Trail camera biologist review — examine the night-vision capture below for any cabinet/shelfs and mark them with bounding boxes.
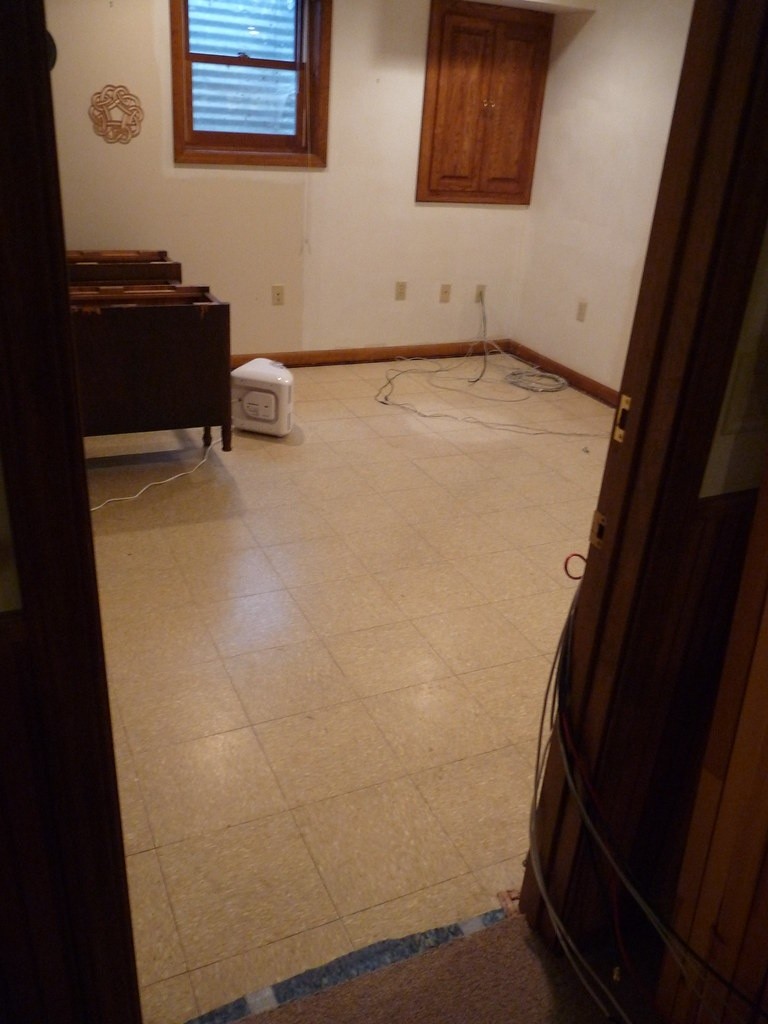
[67,248,234,454]
[414,0,555,206]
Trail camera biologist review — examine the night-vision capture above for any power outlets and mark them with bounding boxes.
[397,282,406,301]
[439,284,451,303]
[273,284,285,306]
[576,300,587,321]
[476,284,486,302]
